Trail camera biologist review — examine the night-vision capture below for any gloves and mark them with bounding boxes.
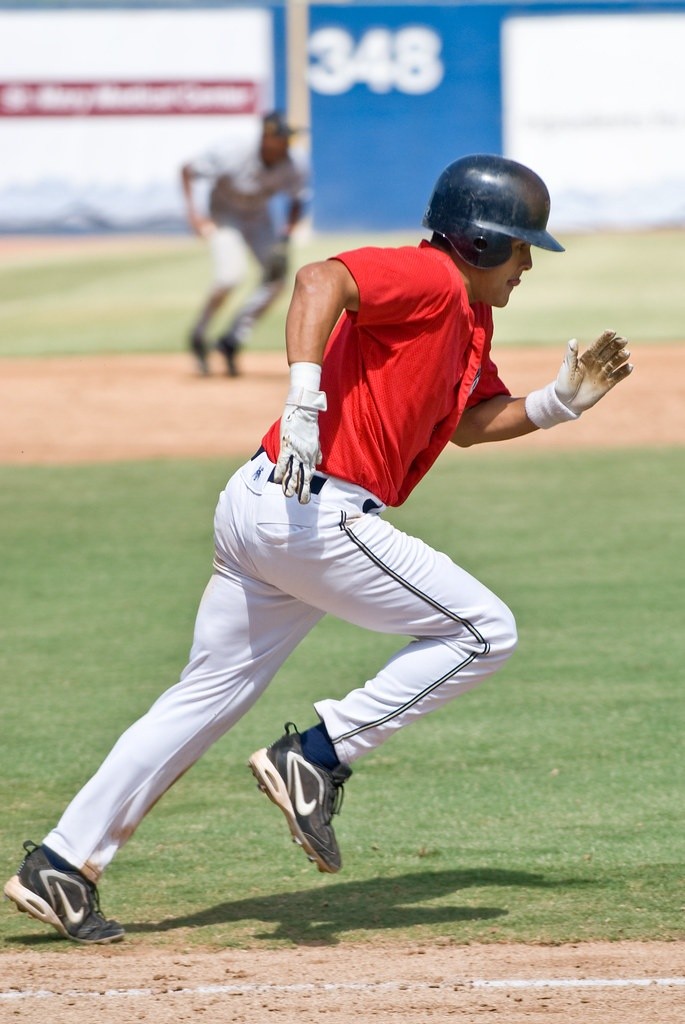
[554,330,633,414]
[274,383,327,504]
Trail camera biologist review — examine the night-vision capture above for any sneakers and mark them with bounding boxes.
[3,840,125,944]
[246,721,352,873]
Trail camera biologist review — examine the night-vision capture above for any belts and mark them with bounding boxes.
[250,446,382,517]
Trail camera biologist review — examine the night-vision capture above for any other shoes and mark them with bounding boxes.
[188,330,212,377]
[220,336,242,376]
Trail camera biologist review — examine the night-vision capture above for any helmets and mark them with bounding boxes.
[421,155,566,269]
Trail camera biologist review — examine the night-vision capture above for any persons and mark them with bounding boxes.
[177,112,312,379]
[0,158,635,948]
